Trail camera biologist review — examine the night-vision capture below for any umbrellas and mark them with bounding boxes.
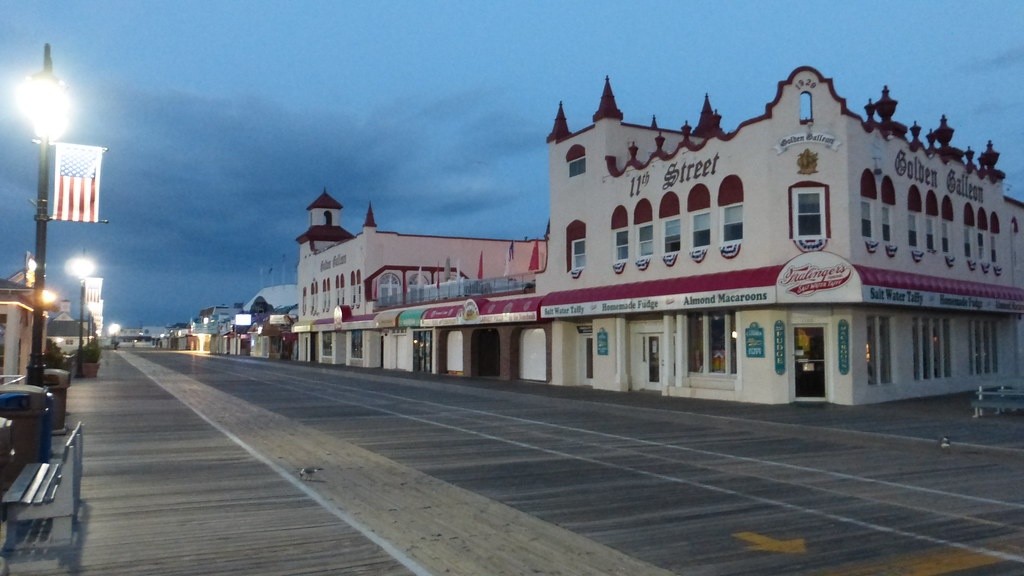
[528,237,539,270]
[371,255,461,301]
[478,250,482,279]
[504,241,513,276]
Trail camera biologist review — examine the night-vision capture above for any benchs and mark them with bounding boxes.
[0,422,84,550]
[970,384,1024,418]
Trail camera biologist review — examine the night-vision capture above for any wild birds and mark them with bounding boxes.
[299,467,324,481]
[940,436,950,448]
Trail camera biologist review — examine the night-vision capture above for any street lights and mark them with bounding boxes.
[73,248,90,380]
[18,44,69,386]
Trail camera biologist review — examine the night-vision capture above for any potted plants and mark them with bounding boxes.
[82,338,101,379]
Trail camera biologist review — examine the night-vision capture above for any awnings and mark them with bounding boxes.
[775,251,1023,314]
[290,296,544,334]
[539,265,783,319]
